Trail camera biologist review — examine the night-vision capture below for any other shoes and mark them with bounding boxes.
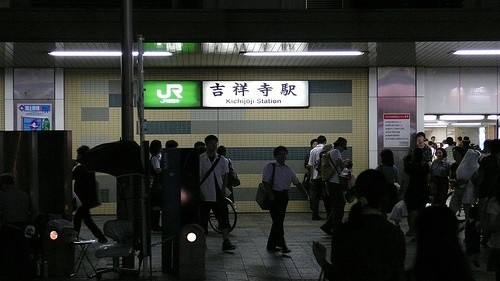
[267,246,280,251]
[406,231,416,237]
[319,225,332,235]
[221,241,236,250]
[282,247,291,253]
[312,216,325,220]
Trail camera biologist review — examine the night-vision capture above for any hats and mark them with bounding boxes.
[334,137,347,150]
[462,136,470,142]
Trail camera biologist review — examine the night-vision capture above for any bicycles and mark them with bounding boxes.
[209,197,237,234]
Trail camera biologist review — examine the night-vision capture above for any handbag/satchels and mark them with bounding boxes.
[389,200,408,223]
[255,183,273,210]
[302,174,311,193]
[461,181,478,204]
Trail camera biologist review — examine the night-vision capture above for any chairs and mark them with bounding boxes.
[73,219,133,281]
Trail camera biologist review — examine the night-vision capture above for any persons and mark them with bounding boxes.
[145,135,239,251]
[72,146,108,243]
[261,146,308,253]
[303,132,500,281]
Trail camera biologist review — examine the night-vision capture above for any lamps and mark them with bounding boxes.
[447,48,500,56]
[46,49,174,58]
[422,114,500,127]
[238,49,369,58]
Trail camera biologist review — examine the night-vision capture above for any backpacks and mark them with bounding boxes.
[321,149,338,180]
